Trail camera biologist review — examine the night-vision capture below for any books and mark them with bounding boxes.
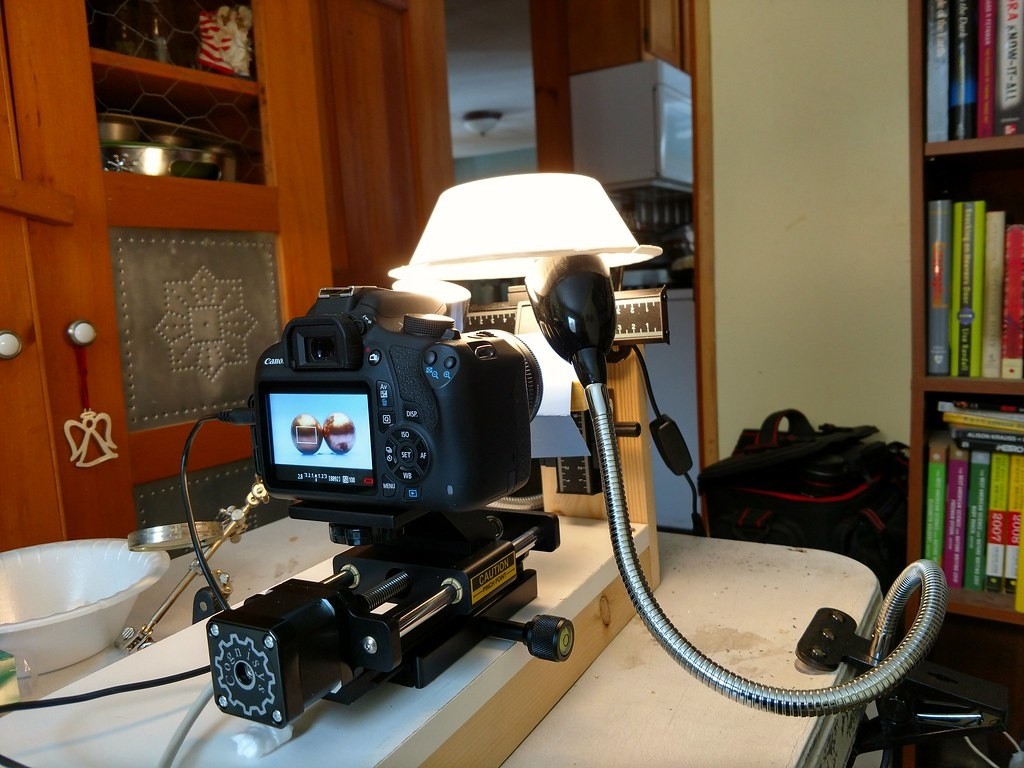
[914,0,1024,618]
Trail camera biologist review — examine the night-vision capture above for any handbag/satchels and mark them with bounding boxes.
[701,403,910,601]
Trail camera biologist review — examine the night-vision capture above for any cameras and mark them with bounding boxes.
[248,287,543,511]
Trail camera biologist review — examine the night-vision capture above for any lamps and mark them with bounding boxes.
[463,110,503,138]
[388,173,664,307]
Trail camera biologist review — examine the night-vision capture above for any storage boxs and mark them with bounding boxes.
[697,408,910,639]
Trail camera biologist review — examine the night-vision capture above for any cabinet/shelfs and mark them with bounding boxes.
[565,0,691,77]
[0,0,454,555]
[905,0,1023,641]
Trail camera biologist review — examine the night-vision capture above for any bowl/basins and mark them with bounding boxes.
[101,108,257,182]
[1,537,173,680]
